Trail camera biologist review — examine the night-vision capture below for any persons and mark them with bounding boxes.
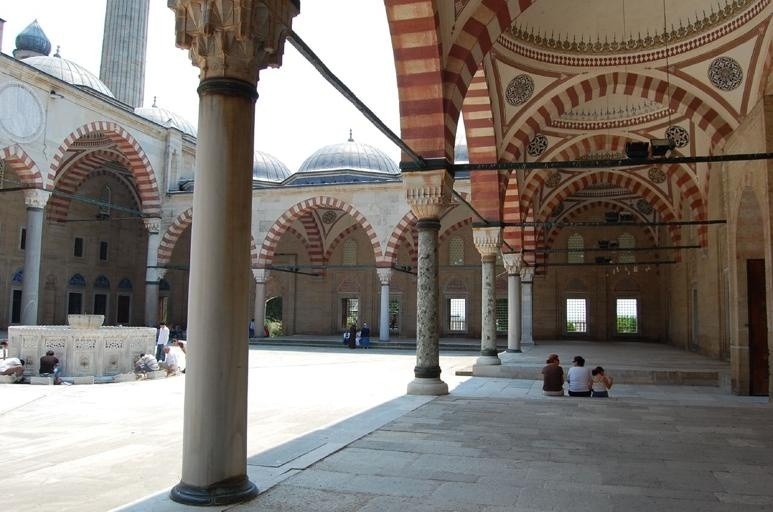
[588,366,615,397]
[541,352,564,396]
[0,342,8,360]
[390,314,397,330]
[343,321,370,348]
[264,323,269,336]
[0,357,26,383]
[249,319,254,338]
[134,321,187,376]
[39,350,62,385]
[566,356,593,397]
